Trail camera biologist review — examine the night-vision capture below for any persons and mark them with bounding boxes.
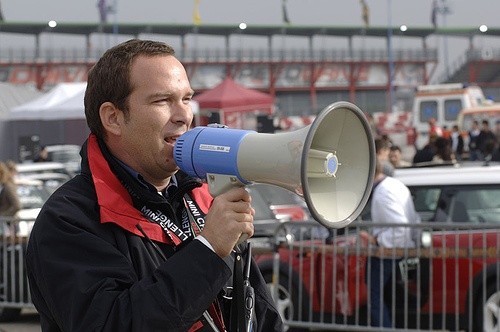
[357,153,423,329]
[34,145,53,161]
[26,38,282,331]
[366,118,499,175]
[0,159,21,237]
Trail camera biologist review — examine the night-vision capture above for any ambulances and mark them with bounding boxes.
[458,102,500,136]
[413,83,488,137]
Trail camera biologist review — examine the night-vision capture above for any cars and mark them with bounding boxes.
[248,182,311,220]
[43,144,82,173]
[0,160,72,320]
[232,187,277,224]
[249,169,500,332]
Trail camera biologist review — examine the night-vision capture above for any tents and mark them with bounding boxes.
[1,78,93,164]
[193,74,273,135]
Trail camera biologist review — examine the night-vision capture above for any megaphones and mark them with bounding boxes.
[173,101,376,230]
